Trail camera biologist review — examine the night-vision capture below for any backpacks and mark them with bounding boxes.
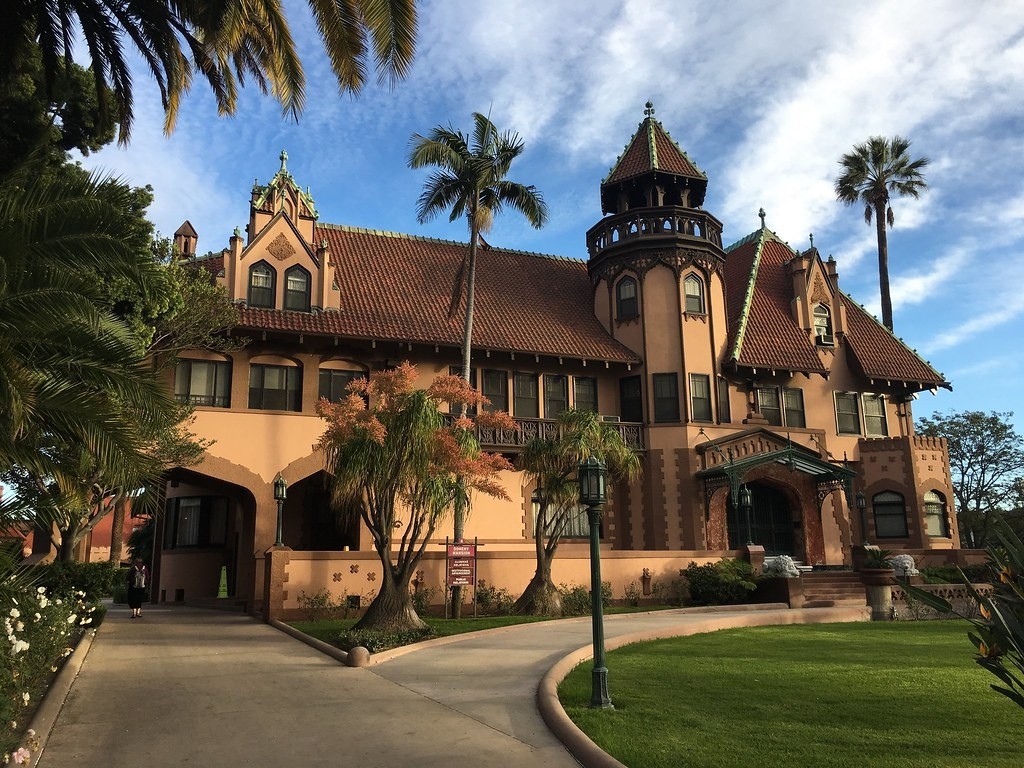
[134,566,146,588]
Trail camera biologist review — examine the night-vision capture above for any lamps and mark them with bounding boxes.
[740,483,752,507]
[785,461,797,473]
[274,477,288,500]
[856,487,867,509]
[578,455,606,505]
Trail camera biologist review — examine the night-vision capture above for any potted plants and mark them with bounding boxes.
[858,549,897,586]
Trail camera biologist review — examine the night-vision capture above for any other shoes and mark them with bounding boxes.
[130,615,136,619]
[136,614,142,617]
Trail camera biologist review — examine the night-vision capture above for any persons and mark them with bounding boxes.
[126,556,150,618]
